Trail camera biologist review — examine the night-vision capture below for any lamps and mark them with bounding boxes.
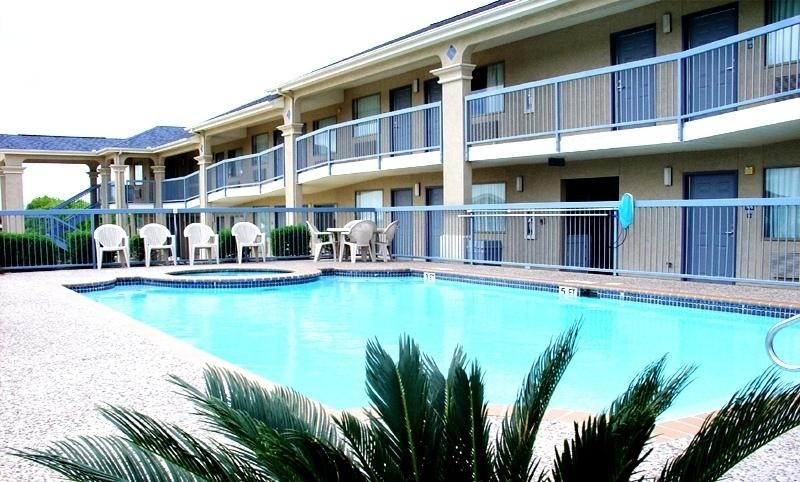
[664,168,672,187]
[515,176,523,191]
[414,183,419,196]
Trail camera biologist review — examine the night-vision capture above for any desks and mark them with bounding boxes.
[327,227,387,262]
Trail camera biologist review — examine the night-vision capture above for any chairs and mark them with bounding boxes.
[338,220,376,263]
[184,223,221,267]
[343,219,362,229]
[231,221,266,265]
[95,224,131,269]
[306,220,337,262]
[139,223,176,269]
[369,220,399,262]
